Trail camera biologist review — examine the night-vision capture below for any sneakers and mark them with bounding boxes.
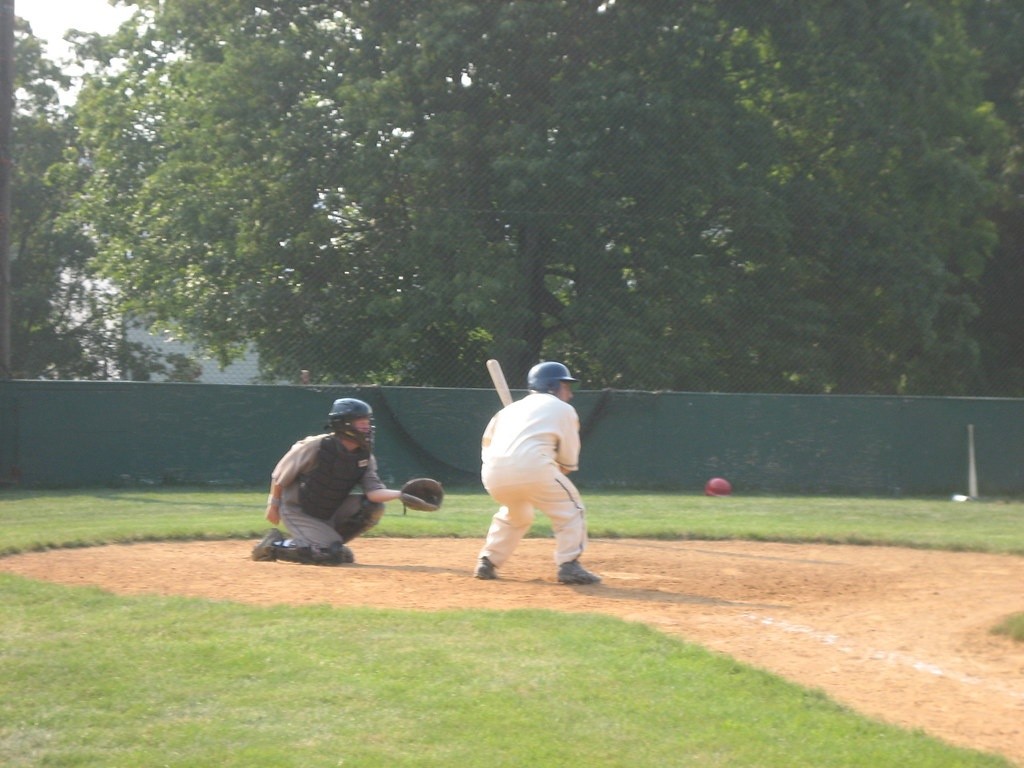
[474,559,497,580]
[331,544,355,564]
[251,529,283,561]
[557,560,602,583]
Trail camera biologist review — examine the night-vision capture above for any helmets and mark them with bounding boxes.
[527,362,579,397]
[329,398,375,456]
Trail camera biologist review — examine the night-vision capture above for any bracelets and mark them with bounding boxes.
[268,494,283,505]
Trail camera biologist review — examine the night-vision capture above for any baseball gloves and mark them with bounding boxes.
[401,479,445,512]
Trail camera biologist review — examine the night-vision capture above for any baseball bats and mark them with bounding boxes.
[486,359,514,408]
[966,423,979,499]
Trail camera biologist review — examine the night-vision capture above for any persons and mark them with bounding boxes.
[474,361,603,583]
[251,397,439,566]
